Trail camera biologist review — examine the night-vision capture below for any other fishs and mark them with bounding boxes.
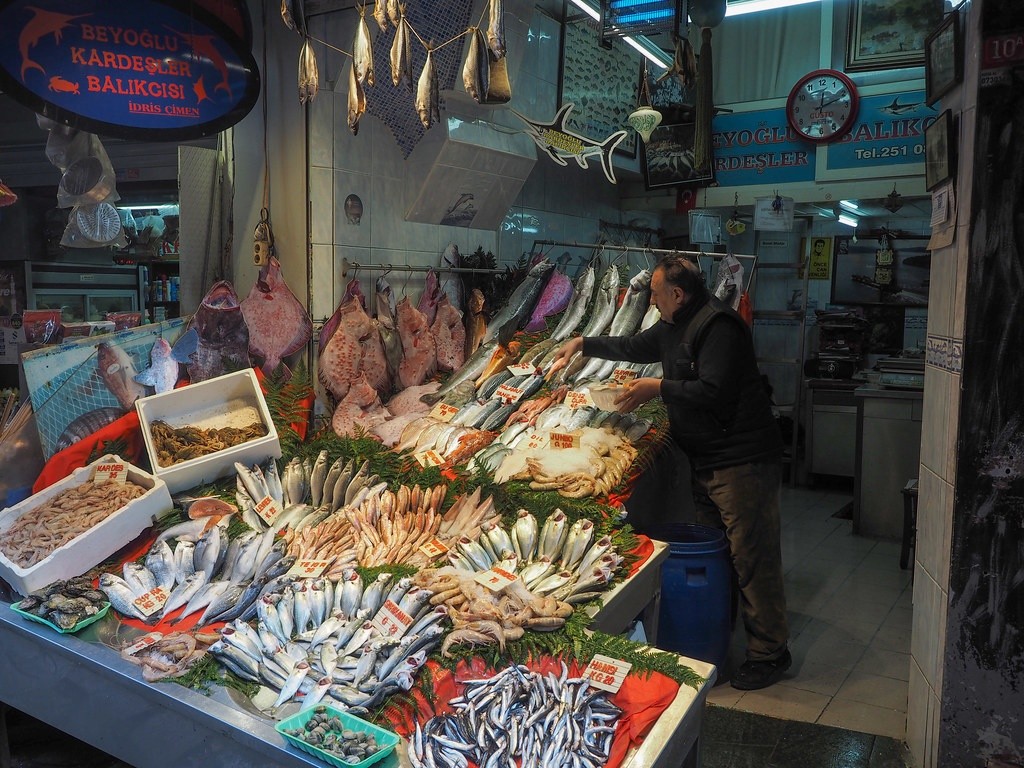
[97,505,297,626]
[298,569,447,715]
[207,619,299,713]
[320,251,745,443]
[402,665,624,768]
[279,0,507,137]
[646,140,707,180]
[233,443,526,567]
[527,508,626,602]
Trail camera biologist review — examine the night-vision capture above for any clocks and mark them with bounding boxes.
[786,68,861,146]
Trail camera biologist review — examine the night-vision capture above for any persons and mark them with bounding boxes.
[555,256,794,691]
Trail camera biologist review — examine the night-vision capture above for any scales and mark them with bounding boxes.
[877,357,925,390]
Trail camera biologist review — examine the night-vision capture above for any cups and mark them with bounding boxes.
[153,279,170,304]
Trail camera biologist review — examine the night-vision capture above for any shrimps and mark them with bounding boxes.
[526,445,638,499]
[111,631,220,683]
[1,471,149,563]
[415,568,574,659]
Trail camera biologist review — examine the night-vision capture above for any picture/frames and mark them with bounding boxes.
[923,107,953,192]
[923,10,962,108]
[637,115,718,193]
[842,0,944,74]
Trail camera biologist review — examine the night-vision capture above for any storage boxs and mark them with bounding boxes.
[0,453,174,600]
[135,366,284,494]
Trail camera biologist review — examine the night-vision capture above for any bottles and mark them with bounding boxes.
[144,267,150,305]
[162,273,168,303]
[170,273,179,302]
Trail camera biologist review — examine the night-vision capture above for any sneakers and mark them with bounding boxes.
[730,648,797,690]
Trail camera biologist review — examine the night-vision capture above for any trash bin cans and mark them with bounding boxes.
[633,520,734,689]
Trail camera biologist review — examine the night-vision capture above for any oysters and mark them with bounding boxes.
[18,576,104,632]
